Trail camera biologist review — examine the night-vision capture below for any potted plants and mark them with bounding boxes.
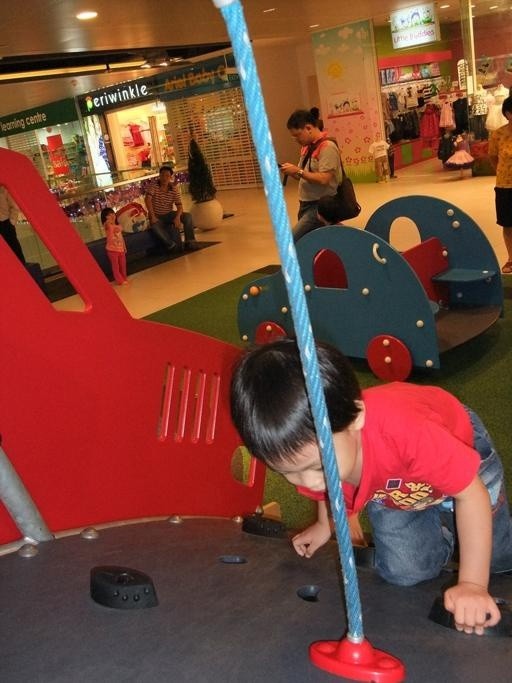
[185,137,224,231]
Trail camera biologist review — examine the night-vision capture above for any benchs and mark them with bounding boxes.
[87,222,182,276]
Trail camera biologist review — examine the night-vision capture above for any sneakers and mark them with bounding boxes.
[171,245,179,253]
[184,241,198,250]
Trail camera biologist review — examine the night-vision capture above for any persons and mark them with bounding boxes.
[311,107,323,132]
[488,97,512,274]
[227,330,511,636]
[1,185,26,267]
[317,196,344,225]
[143,165,198,253]
[368,134,391,182]
[385,137,397,179]
[482,83,512,132]
[277,108,344,247]
[100,206,130,286]
[446,134,475,169]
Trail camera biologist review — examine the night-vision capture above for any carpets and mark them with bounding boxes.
[44,239,221,306]
[137,263,511,541]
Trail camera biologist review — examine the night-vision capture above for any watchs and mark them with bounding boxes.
[294,168,304,180]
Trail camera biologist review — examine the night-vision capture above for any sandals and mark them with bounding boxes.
[502,261,512,273]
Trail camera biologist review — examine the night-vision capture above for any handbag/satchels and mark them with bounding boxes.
[332,177,361,219]
